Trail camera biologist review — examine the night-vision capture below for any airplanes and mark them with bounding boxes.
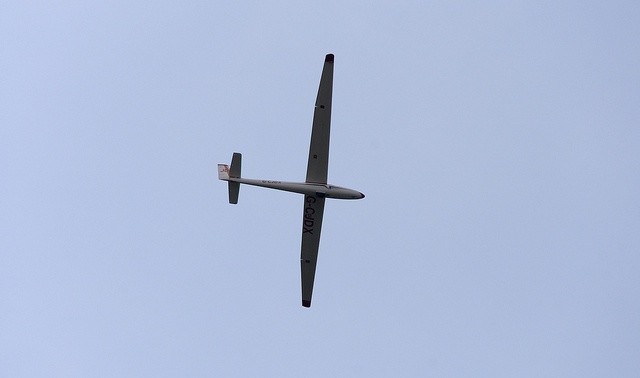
[217,54,366,308]
